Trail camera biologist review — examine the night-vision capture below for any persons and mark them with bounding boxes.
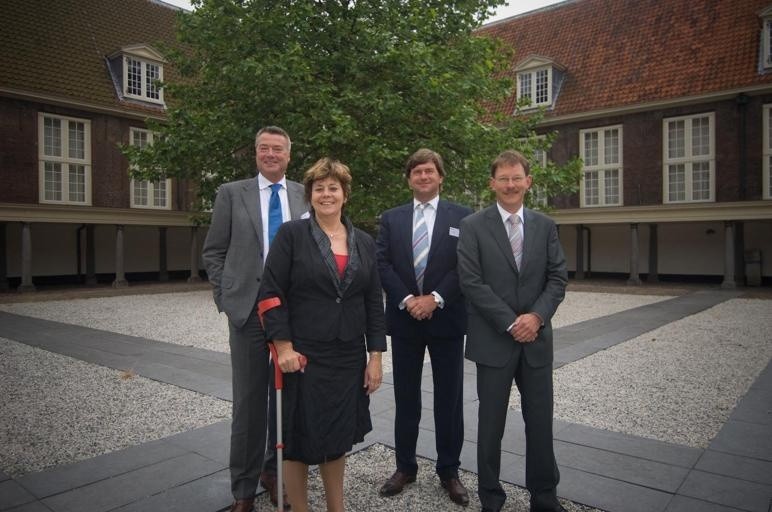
[375,148,472,508]
[454,150,569,511]
[200,125,311,510]
[256,159,385,510]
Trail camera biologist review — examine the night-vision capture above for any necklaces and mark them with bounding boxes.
[321,223,340,240]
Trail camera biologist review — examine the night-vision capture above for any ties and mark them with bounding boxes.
[412,203,431,294]
[268,184,282,248]
[508,214,523,272]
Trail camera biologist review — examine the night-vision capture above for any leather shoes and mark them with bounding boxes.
[230,478,291,512]
[380,471,416,496]
[441,477,469,506]
